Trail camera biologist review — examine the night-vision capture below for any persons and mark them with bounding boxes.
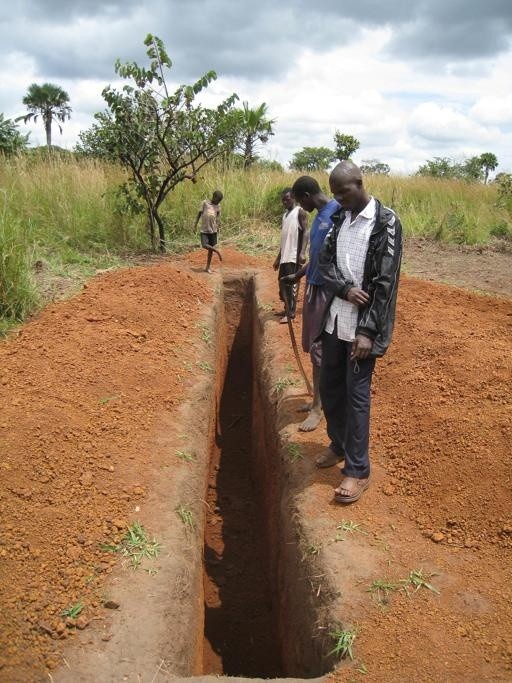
[315,162,403,503]
[279,175,343,432]
[273,187,309,323]
[193,190,224,274]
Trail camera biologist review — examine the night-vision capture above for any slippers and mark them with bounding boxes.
[316,449,346,468]
[332,475,371,503]
